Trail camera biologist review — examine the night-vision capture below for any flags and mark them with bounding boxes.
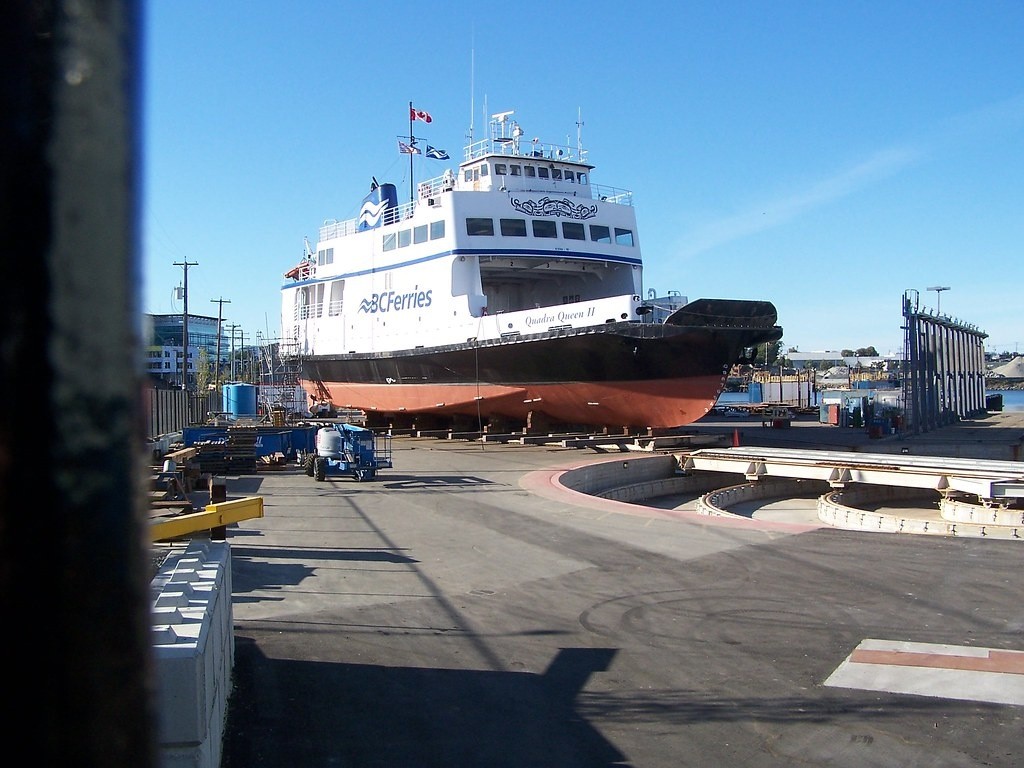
[425,144,450,161]
[397,141,422,155]
[411,108,433,125]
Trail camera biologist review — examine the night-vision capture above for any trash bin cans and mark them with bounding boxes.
[828,403,840,424]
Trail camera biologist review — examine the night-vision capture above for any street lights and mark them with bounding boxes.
[924,286,951,313]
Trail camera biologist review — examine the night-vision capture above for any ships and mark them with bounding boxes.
[274,99,787,430]
